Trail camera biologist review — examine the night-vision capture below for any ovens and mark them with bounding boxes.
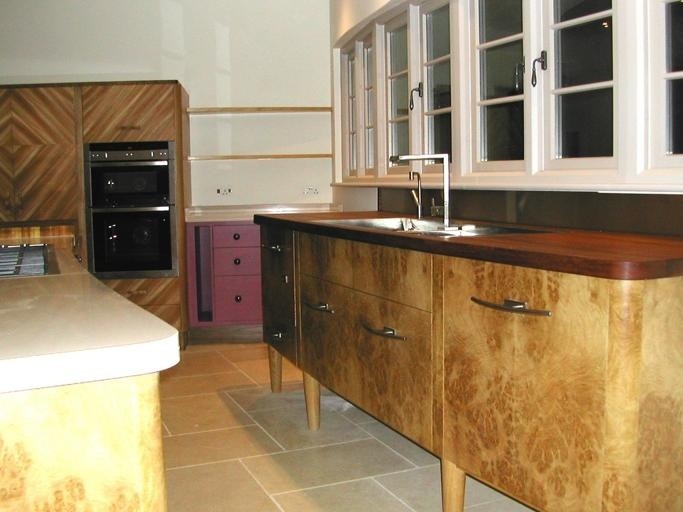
[83,140,180,279]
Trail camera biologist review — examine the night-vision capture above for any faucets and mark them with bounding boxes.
[390,153,450,230]
[409,172,422,219]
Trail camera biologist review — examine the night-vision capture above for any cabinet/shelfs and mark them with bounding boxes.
[80,82,189,143]
[0,82,76,229]
[260,226,297,367]
[97,277,181,334]
[186,221,260,328]
[185,105,333,161]
[443,256,682,510]
[296,231,433,454]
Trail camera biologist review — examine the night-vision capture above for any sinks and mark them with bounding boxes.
[418,224,551,236]
[318,217,442,229]
